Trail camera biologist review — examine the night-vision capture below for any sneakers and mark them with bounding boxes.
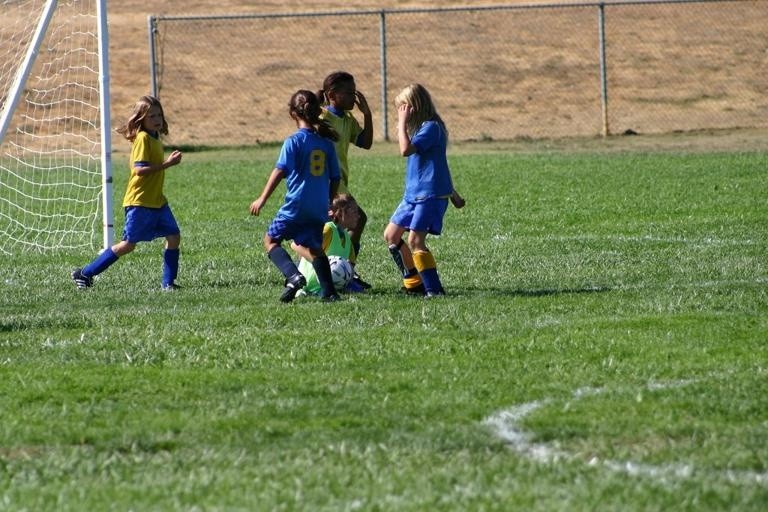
[279,272,371,302]
[162,284,183,289]
[398,283,445,299]
[71,269,93,290]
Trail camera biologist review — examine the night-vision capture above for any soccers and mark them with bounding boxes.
[316,255,352,290]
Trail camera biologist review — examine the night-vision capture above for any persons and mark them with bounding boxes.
[290,192,361,298]
[314,72,373,290]
[71,96,184,291]
[383,83,465,298]
[250,90,342,303]
